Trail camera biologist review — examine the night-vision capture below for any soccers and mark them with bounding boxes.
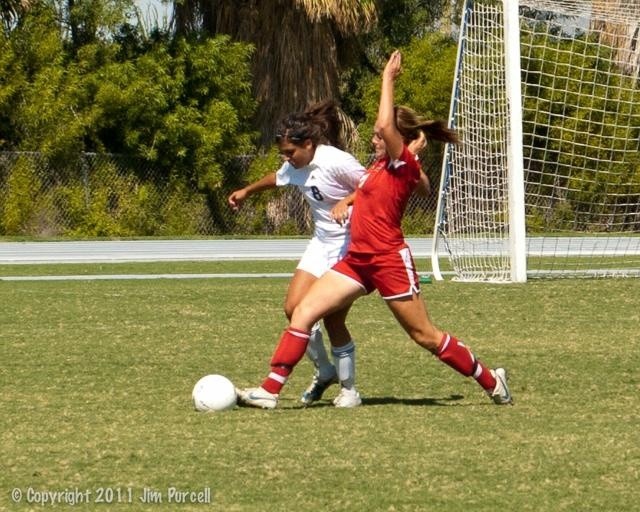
[191,375,237,414]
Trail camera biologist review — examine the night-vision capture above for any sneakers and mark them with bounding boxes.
[300,365,362,409]
[236,386,279,410]
[491,368,511,405]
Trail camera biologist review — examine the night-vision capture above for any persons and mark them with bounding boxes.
[234,46,515,416]
[225,98,365,411]
